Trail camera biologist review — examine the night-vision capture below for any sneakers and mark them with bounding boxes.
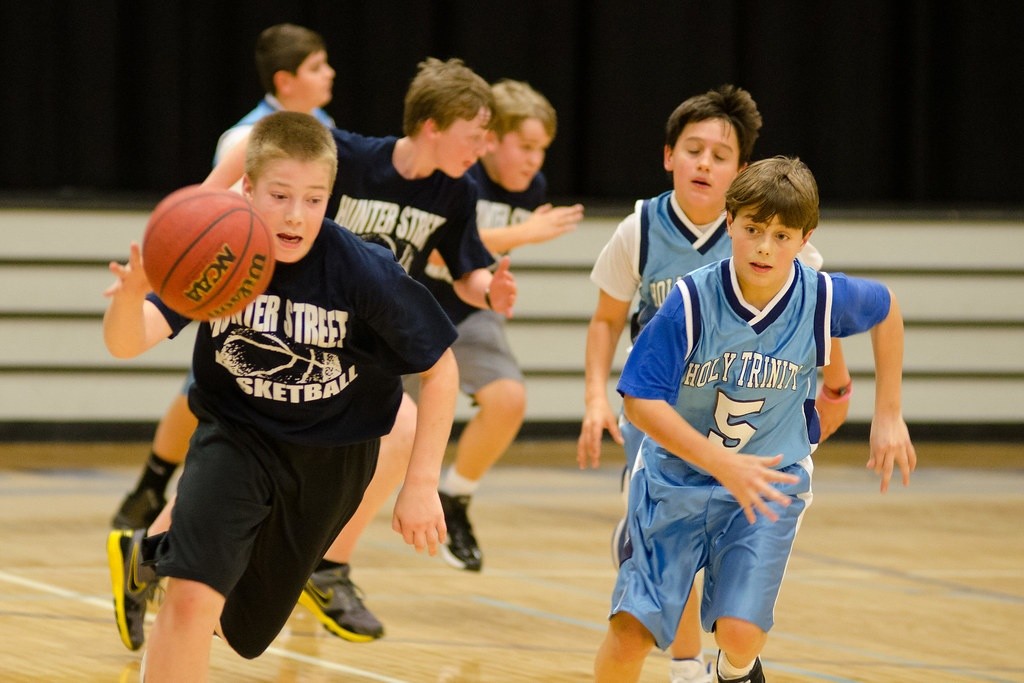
[437,488,482,571]
[106,529,167,653]
[297,564,385,644]
[112,489,166,538]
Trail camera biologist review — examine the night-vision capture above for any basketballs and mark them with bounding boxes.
[140,184,277,322]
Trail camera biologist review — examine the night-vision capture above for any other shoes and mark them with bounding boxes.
[668,658,715,683]
[612,465,633,570]
[715,649,766,683]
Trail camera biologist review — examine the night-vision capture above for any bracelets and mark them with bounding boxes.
[822,380,851,403]
[485,288,491,309]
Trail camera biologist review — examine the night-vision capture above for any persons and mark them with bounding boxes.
[102,114,460,683]
[113,24,337,530]
[574,87,852,683]
[106,61,516,651]
[592,159,917,683]
[404,82,583,572]
